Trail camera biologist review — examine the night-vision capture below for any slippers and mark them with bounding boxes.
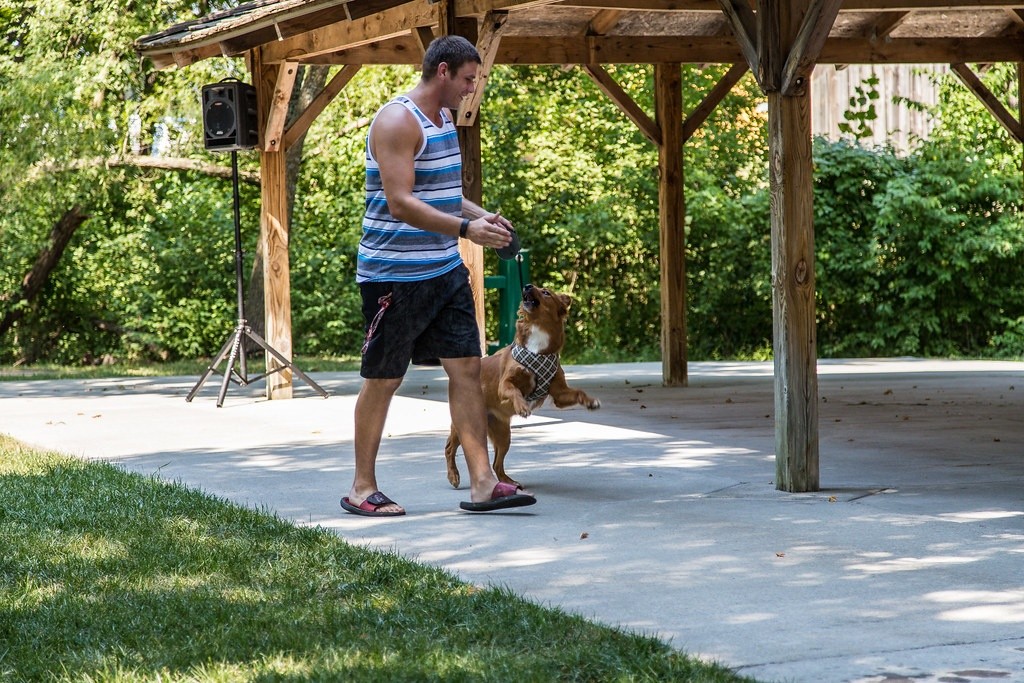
[460,481,537,511]
[340,492,406,517]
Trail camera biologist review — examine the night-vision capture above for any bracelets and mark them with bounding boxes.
[459,218,471,238]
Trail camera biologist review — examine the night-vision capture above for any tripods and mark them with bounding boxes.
[185,148,331,407]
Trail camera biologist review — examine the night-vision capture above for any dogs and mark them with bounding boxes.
[445,283,601,489]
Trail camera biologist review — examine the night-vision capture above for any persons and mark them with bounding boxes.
[340,36,537,516]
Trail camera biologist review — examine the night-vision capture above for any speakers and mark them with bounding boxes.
[202,83,259,149]
